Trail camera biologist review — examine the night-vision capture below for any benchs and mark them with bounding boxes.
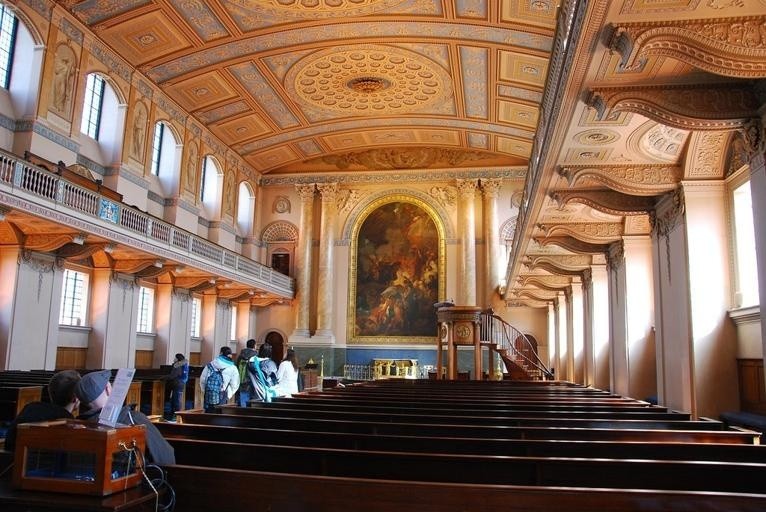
[0,366,766,512]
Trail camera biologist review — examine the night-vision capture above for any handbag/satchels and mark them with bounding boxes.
[298,374,304,392]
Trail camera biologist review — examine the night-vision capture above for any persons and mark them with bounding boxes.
[5,368,80,450]
[237,339,258,406]
[199,346,241,408]
[276,348,301,397]
[166,353,189,413]
[246,344,279,401]
[74,370,177,466]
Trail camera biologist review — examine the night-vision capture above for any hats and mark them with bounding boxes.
[75,369,112,404]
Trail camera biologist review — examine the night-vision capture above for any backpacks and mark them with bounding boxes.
[238,354,256,392]
[204,363,231,412]
[175,363,188,384]
